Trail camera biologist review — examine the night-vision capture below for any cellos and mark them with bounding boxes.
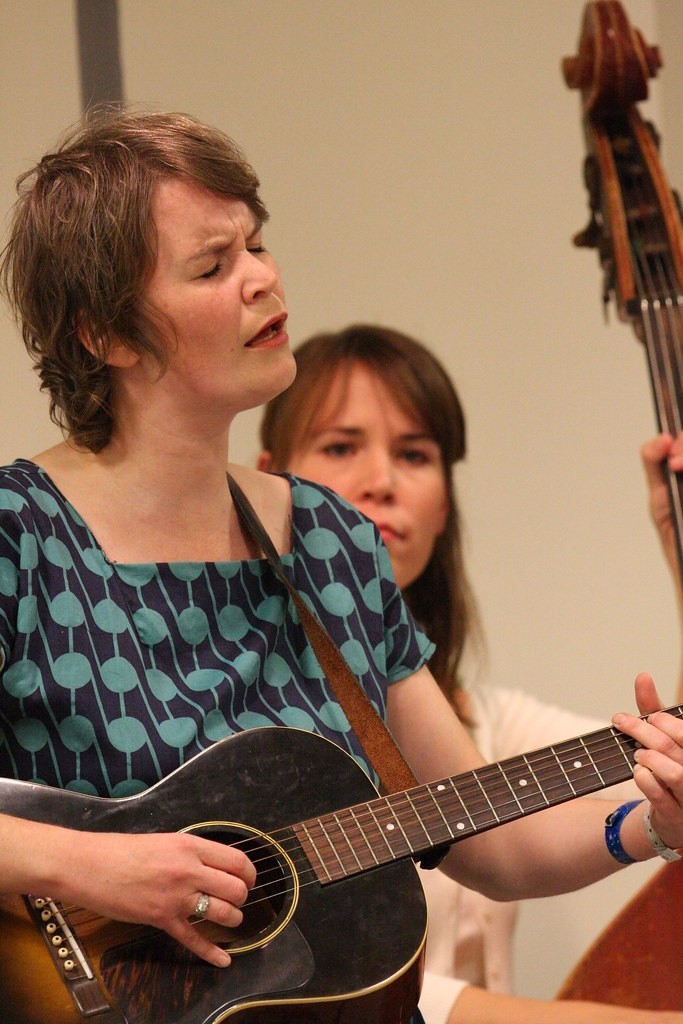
[553,0,683,1013]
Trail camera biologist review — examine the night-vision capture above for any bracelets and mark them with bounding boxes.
[602,796,648,864]
[642,804,682,863]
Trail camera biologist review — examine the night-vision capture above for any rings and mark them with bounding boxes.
[193,893,209,918]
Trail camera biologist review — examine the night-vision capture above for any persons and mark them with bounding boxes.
[255,326,683,1024]
[0,110,683,1024]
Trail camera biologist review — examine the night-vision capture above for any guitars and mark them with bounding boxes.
[1,700,682,1024]
